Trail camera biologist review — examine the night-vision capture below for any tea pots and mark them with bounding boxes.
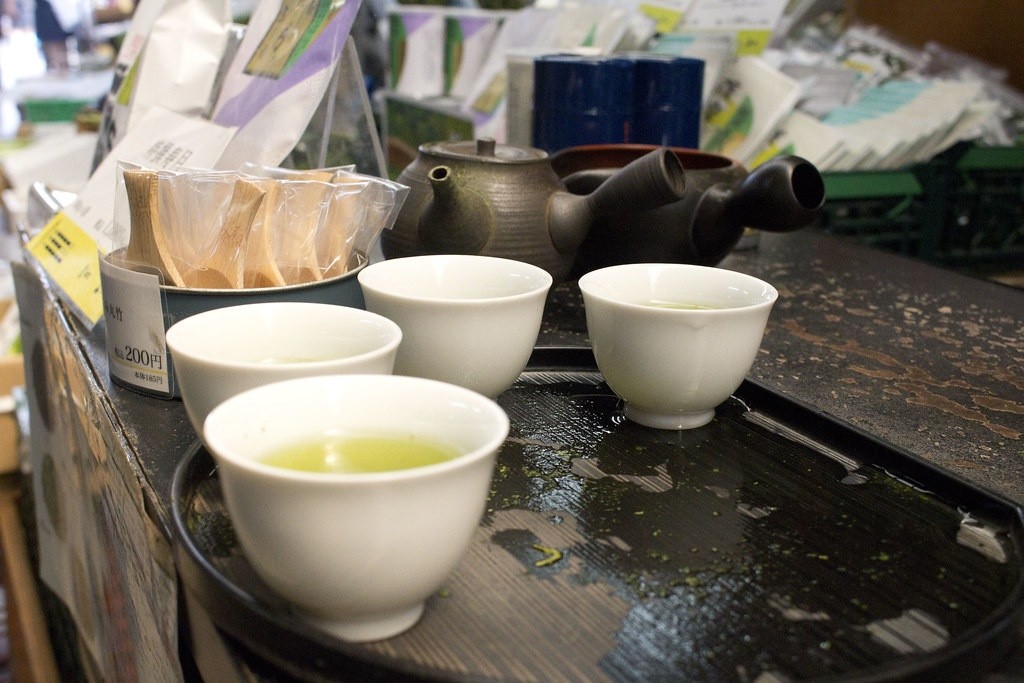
[380,136,688,286]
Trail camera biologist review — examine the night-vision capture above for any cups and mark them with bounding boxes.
[356,255,553,405]
[165,302,403,457]
[505,52,704,157]
[204,375,510,643]
[577,262,779,431]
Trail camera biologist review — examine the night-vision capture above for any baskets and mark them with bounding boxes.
[817,144,1024,271]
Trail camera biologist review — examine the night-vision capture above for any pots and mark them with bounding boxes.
[555,145,826,269]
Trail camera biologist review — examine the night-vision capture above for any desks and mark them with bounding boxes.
[14,228,1024,683]
